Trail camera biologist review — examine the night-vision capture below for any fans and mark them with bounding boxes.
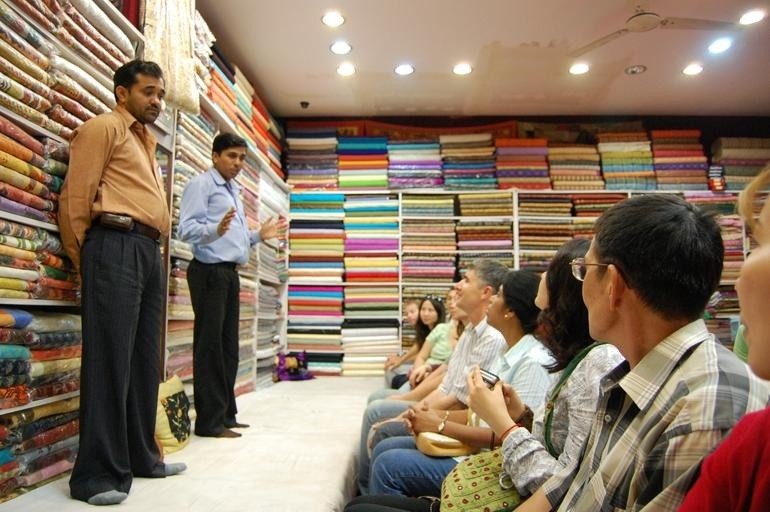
[568,0,747,57]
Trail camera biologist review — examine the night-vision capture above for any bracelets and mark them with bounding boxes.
[518,406,534,427]
[444,409,450,420]
[439,419,445,433]
[500,424,519,442]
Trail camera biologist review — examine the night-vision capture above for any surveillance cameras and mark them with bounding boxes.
[300,102,309,109]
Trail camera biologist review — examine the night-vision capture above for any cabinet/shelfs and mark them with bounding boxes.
[288,189,770,377]
[0,0,287,503]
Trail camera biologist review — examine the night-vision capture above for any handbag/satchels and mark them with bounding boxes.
[439,446,529,511]
[416,431,481,458]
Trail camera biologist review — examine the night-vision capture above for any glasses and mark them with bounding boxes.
[425,294,442,302]
[570,257,611,282]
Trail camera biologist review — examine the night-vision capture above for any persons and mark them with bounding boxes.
[368,295,470,403]
[370,270,559,499]
[343,237,626,511]
[674,161,769,511]
[514,193,770,512]
[58,59,187,506]
[177,133,290,439]
[357,259,510,494]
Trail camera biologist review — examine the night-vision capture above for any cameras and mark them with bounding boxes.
[479,367,499,385]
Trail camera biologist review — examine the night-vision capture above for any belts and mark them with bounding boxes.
[91,213,164,246]
[215,260,242,271]
